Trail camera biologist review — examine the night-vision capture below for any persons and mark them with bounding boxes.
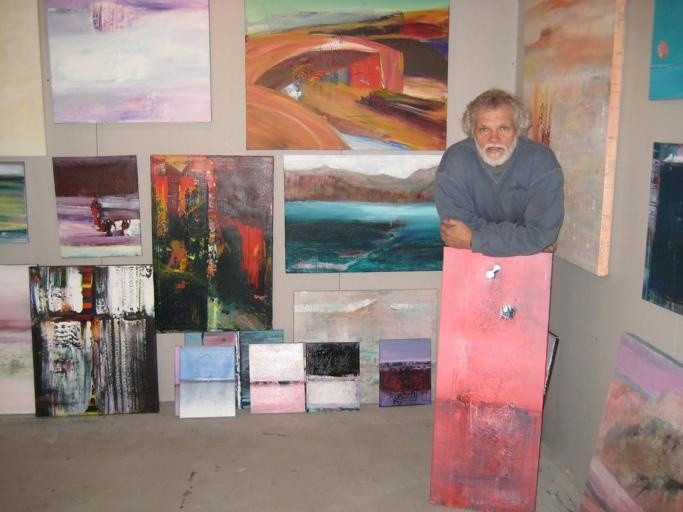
[433,89,564,257]
